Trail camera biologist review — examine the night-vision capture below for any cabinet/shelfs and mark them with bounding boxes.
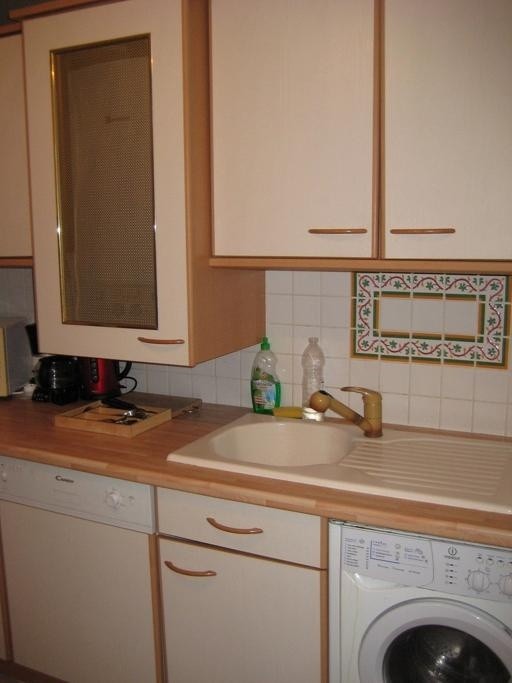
[8,0,265,367]
[208,0,512,275]
[156,487,331,683]
[1,454,164,682]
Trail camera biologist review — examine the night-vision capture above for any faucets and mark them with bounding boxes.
[306,386,384,438]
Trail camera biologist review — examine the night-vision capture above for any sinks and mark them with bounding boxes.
[167,410,353,484]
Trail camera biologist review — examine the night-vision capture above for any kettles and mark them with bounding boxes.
[77,357,132,401]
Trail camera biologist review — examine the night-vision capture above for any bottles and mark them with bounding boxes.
[301,336,325,409]
[251,336,282,415]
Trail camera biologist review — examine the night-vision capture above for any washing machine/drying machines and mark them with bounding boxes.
[328,517,512,683]
